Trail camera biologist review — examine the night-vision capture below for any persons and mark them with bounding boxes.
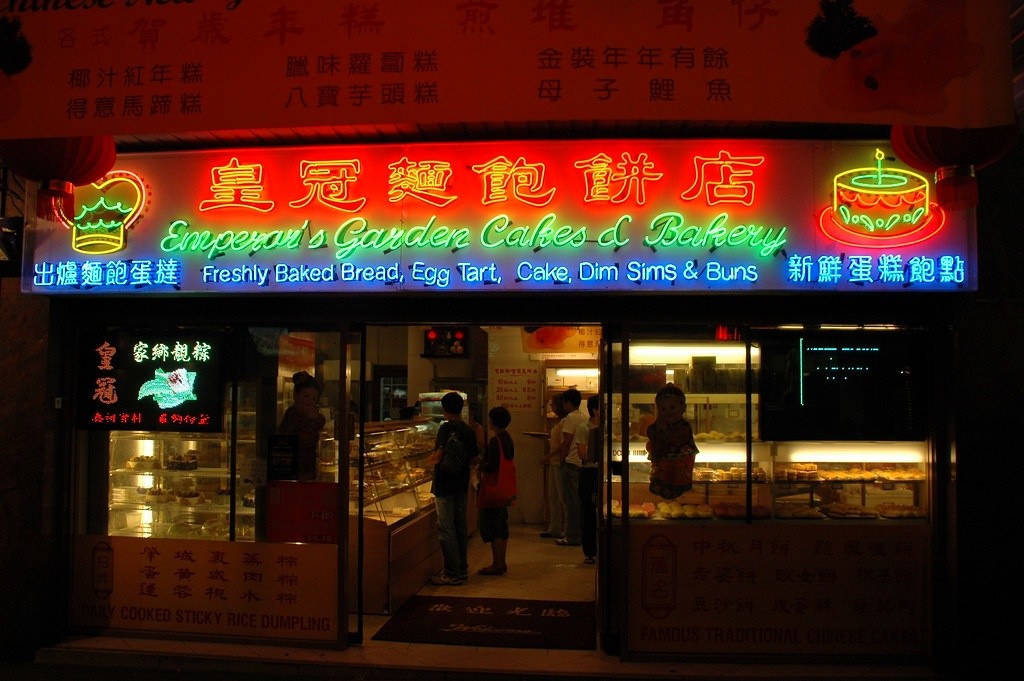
[538,389,606,565]
[425,391,480,585]
[475,407,515,576]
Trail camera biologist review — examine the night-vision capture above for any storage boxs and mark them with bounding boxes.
[616,365,667,393]
[675,357,759,394]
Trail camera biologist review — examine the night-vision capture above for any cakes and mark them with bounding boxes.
[125,452,256,508]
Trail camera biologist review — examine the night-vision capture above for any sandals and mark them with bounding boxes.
[584,554,597,564]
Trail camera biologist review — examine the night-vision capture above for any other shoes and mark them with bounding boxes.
[555,538,581,546]
[540,531,561,538]
[430,570,468,585]
[479,563,507,576]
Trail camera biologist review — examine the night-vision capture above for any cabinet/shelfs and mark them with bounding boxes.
[543,359,762,531]
[108,417,486,615]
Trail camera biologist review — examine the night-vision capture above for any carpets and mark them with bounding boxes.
[371,595,597,651]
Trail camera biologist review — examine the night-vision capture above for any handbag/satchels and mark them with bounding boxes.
[476,437,517,509]
[439,421,467,473]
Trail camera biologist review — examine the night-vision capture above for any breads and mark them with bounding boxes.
[774,463,926,520]
[601,498,770,518]
[349,438,437,516]
[692,431,760,442]
[691,465,767,482]
[611,432,648,442]
